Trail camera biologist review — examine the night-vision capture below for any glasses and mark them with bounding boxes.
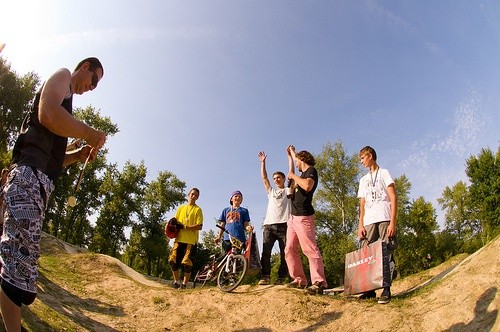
[92,67,99,88]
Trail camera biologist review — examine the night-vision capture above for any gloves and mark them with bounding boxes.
[174,221,186,230]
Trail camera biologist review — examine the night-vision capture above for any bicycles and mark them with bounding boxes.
[193,224,248,292]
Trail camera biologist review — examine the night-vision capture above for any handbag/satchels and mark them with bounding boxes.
[244,229,263,276]
[344,236,390,295]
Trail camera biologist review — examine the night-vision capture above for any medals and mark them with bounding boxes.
[68,196,77,207]
[372,188,377,193]
[185,218,188,221]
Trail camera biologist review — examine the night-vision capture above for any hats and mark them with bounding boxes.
[229,190,243,205]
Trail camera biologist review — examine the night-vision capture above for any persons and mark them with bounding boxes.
[258,145,295,285]
[0,57,106,332]
[168,188,204,290]
[216,190,254,286]
[285,147,328,290]
[357,146,397,304]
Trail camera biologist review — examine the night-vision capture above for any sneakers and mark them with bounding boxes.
[273,278,284,285]
[258,276,271,285]
[178,284,186,290]
[305,281,323,294]
[171,283,179,287]
[285,280,306,289]
[378,290,391,304]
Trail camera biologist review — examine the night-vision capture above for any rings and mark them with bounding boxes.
[100,145,102,148]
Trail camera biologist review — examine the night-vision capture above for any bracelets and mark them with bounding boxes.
[185,225,187,230]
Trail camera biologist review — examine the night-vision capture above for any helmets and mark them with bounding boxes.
[166,217,180,238]
